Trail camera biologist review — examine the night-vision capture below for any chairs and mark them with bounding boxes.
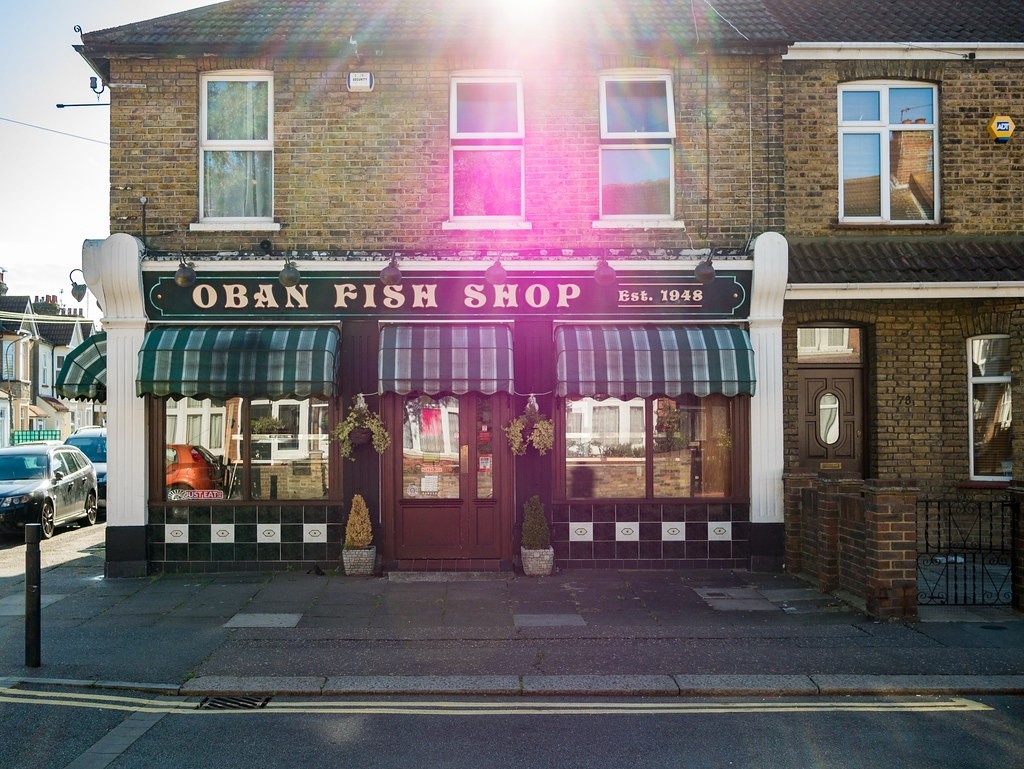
[30,456,46,478]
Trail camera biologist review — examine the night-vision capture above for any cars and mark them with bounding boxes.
[165,445,224,520]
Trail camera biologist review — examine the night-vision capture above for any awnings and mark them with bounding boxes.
[377,324,515,396]
[135,325,339,400]
[55,332,107,403]
[554,324,756,398]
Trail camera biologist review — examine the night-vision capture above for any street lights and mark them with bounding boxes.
[6,333,33,446]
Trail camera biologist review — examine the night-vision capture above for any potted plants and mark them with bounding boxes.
[254,417,290,435]
[342,494,376,575]
[521,495,554,576]
[329,395,391,458]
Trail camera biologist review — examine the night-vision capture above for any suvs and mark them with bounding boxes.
[63,425,109,523]
[0,440,100,541]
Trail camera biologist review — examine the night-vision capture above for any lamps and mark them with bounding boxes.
[279,248,300,287]
[69,269,87,301]
[694,247,716,285]
[175,250,197,288]
[595,251,616,285]
[90,76,105,99]
[501,397,555,456]
[485,253,506,285]
[380,250,402,283]
